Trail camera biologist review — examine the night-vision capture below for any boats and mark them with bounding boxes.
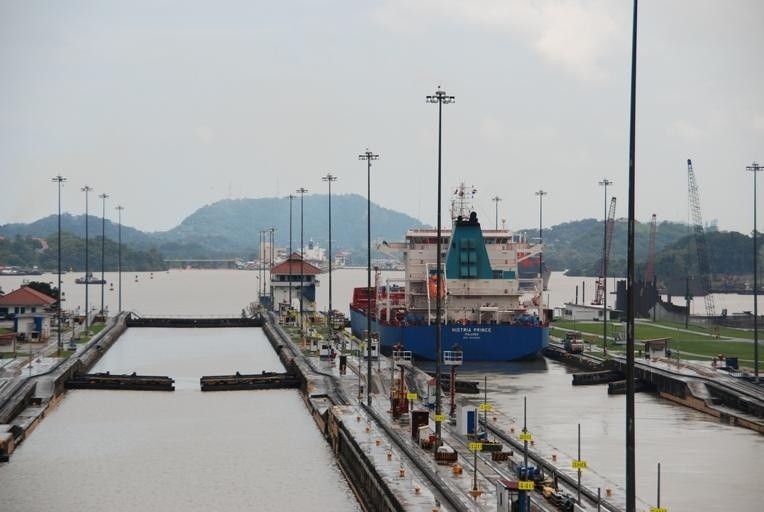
[343,183,556,370]
[1,265,68,278]
[74,270,110,286]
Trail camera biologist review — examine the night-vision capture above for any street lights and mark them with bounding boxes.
[741,160,762,384]
[49,174,68,348]
[482,180,614,355]
[257,166,336,341]
[114,206,124,315]
[97,193,107,321]
[425,83,462,456]
[80,184,93,337]
[355,148,385,406]
